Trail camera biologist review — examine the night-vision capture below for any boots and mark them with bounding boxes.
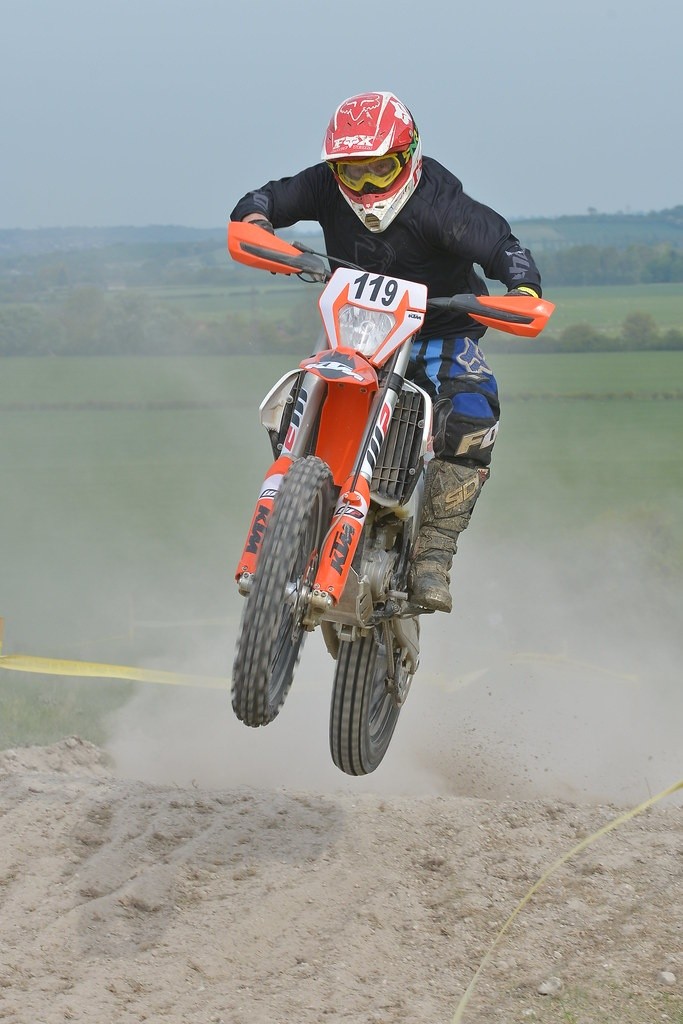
[405,457,487,613]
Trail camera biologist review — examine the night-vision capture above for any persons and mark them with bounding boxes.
[230,91,542,613]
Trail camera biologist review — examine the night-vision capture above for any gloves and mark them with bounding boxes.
[248,219,291,276]
[504,288,535,297]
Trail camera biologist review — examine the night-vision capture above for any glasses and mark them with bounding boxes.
[325,106,419,197]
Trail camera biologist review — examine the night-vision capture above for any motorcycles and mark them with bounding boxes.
[225,230,554,776]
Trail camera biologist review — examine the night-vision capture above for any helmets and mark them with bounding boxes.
[320,91,421,233]
